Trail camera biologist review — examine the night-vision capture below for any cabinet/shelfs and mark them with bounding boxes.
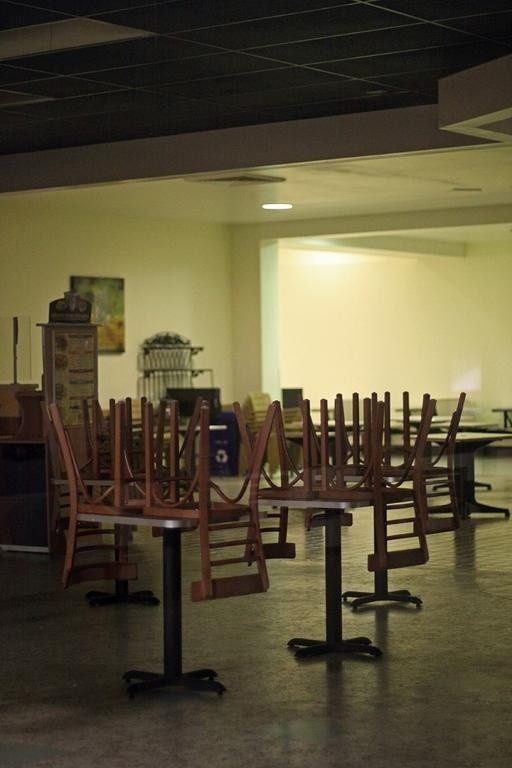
[137,330,214,406]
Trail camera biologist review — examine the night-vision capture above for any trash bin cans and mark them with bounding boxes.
[208,412,240,477]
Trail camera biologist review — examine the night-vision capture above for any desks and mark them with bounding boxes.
[76,512,268,700]
[314,474,462,612]
[407,429,509,521]
[392,408,508,492]
[256,497,415,657]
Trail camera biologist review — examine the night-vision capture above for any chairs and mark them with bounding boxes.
[41,392,466,603]
